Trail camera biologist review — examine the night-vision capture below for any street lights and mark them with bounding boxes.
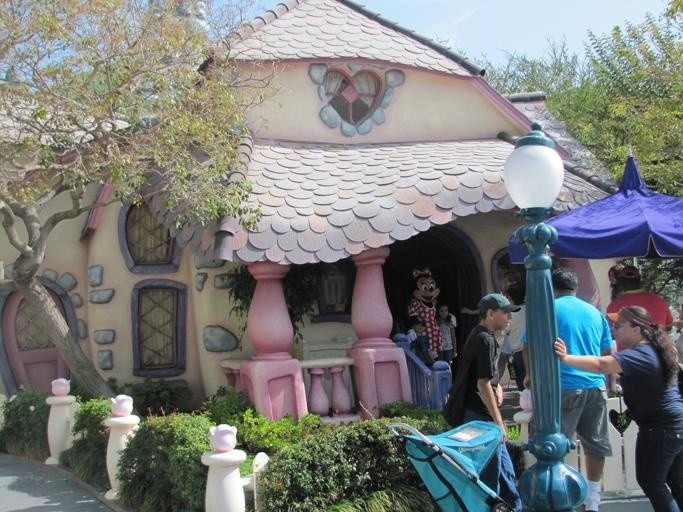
[497,117,591,511]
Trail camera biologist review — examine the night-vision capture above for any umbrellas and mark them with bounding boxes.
[506,144,682,262]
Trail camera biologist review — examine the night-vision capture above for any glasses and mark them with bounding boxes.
[613,320,623,328]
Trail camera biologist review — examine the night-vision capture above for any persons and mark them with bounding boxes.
[553,305,683,512]
[520,267,614,512]
[409,315,435,370]
[435,300,458,366]
[461,263,683,397]
[459,293,525,512]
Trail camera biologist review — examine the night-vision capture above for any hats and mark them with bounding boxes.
[477,293,521,314]
[617,266,641,278]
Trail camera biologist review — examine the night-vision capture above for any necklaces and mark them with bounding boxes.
[635,336,645,345]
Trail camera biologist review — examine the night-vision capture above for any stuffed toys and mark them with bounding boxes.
[406,266,456,363]
